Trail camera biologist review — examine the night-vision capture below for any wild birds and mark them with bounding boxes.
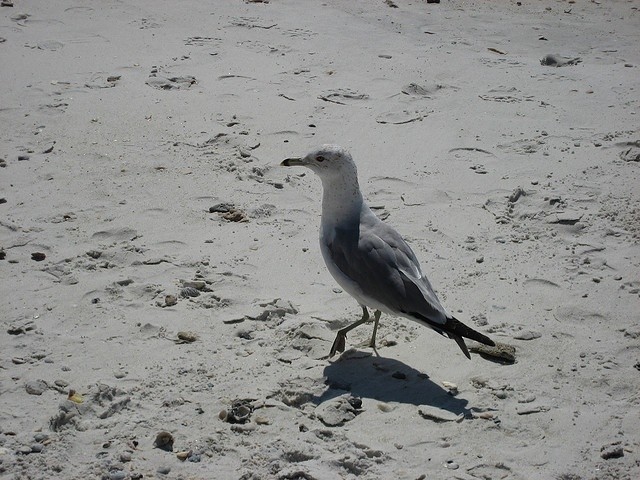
[279,144,496,361]
